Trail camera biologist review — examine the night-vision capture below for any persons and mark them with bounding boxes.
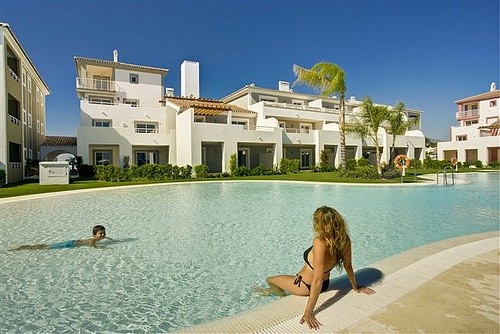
[261,206,376,330]
[9,225,119,249]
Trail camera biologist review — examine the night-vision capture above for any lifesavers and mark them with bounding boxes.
[395,155,410,171]
[451,157,457,166]
